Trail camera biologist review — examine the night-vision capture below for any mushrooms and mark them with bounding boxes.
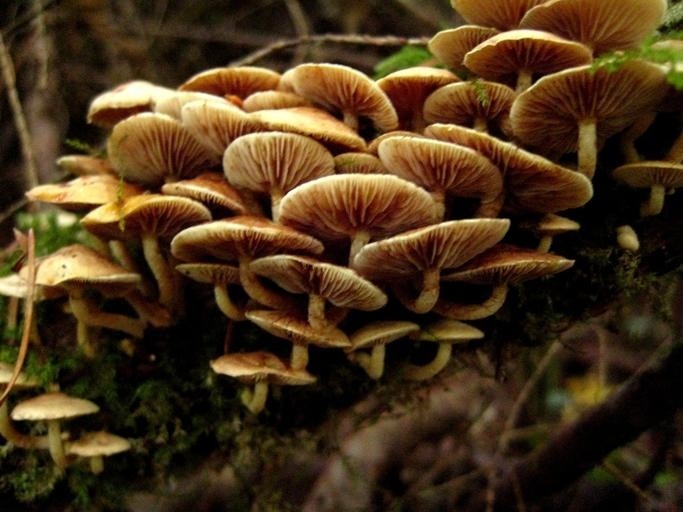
[0,1,681,479]
